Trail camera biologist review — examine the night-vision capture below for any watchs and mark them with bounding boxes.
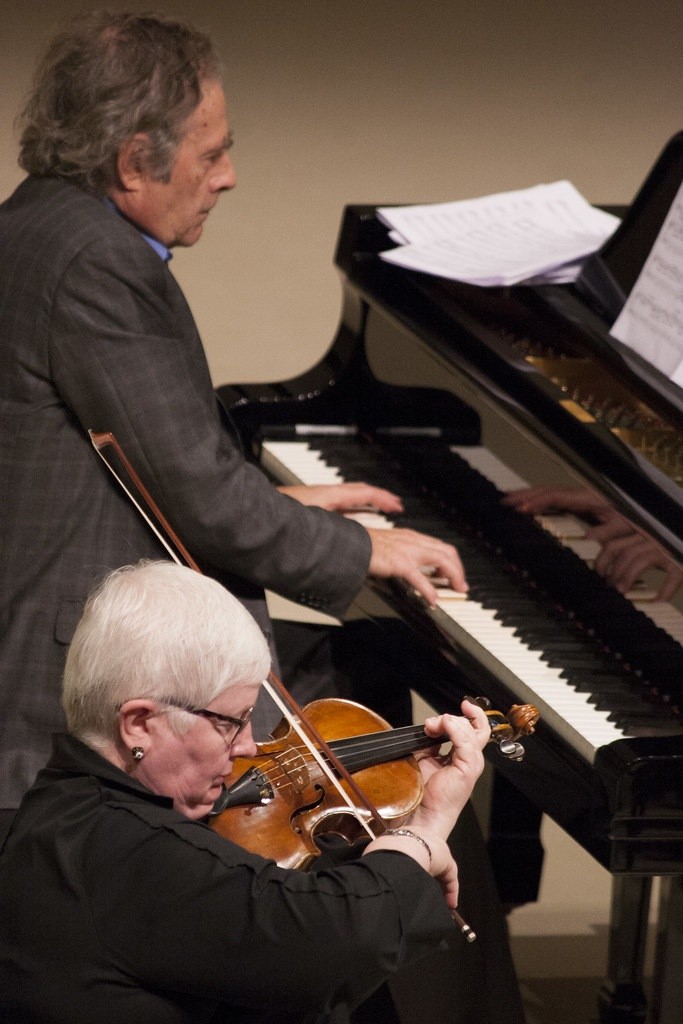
[377,827,432,868]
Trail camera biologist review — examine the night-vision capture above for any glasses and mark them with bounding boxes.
[163,698,256,745]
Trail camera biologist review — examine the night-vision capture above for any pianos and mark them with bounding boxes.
[214,125,683,1022]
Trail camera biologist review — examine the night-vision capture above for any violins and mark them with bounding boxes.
[206,695,541,871]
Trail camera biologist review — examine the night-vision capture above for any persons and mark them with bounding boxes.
[0,14,548,1023]
[502,481,682,600]
[1,559,491,1024]
